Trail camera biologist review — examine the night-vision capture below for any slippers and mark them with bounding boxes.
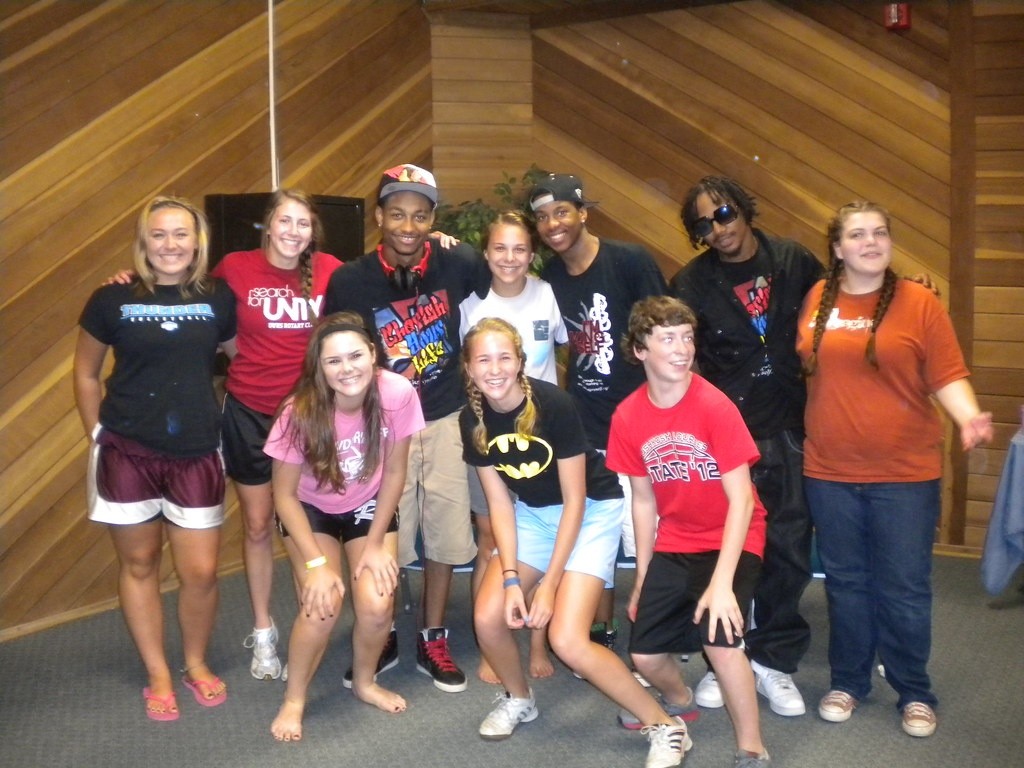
[142,686,180,721]
[180,673,228,707]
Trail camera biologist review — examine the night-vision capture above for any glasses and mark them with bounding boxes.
[691,204,740,238]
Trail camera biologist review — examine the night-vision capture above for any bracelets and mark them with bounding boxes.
[503,577,521,589]
[503,570,518,575]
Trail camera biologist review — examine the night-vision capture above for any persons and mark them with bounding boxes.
[458,317,693,768]
[323,164,491,690]
[306,556,328,569]
[529,173,668,688]
[101,188,460,682]
[263,312,426,743]
[73,196,238,721]
[605,295,772,768]
[797,201,994,733]
[669,176,941,717]
[458,209,570,684]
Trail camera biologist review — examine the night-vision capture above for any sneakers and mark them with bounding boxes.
[901,701,937,737]
[818,689,858,723]
[616,686,702,730]
[342,628,400,689]
[416,627,468,693]
[243,619,282,681]
[639,715,694,768]
[734,748,772,768]
[478,685,539,741]
[695,671,725,708]
[751,658,806,717]
[280,662,288,681]
[572,616,620,680]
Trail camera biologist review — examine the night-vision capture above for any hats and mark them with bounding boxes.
[526,173,600,212]
[377,164,439,210]
